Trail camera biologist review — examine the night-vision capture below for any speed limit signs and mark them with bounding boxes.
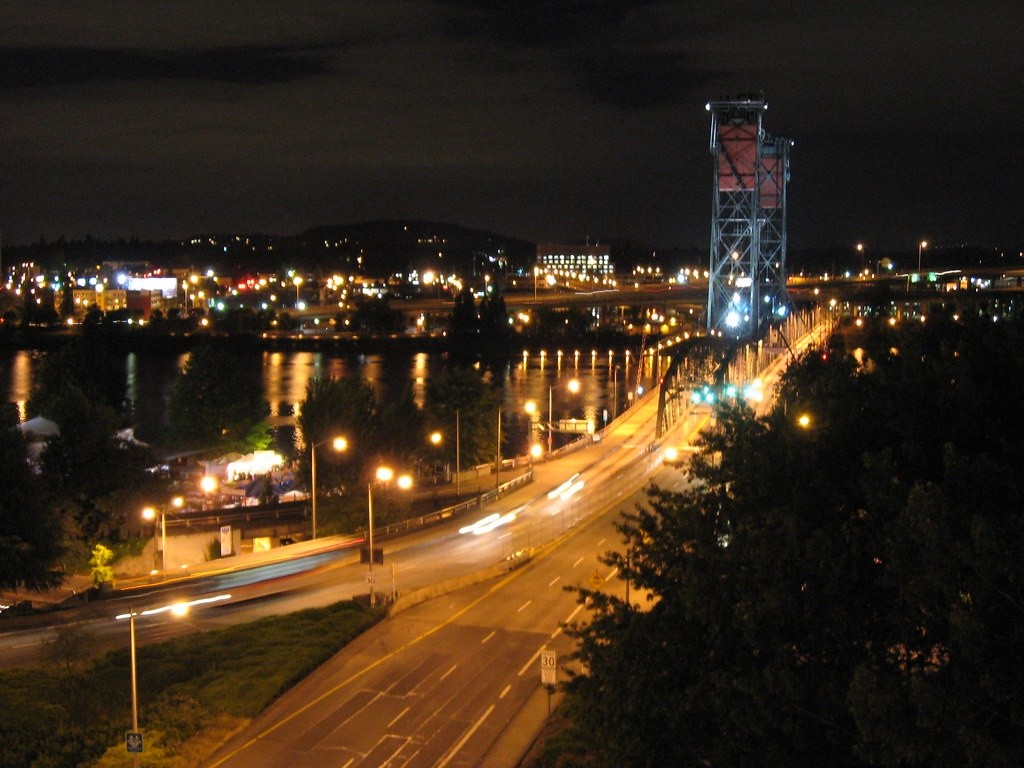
[541,650,556,670]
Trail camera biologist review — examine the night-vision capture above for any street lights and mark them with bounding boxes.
[182,280,189,313]
[366,467,395,611]
[293,277,302,311]
[142,504,166,575]
[534,267,540,301]
[494,399,537,486]
[918,241,927,273]
[546,378,580,456]
[128,599,191,768]
[311,436,347,540]
